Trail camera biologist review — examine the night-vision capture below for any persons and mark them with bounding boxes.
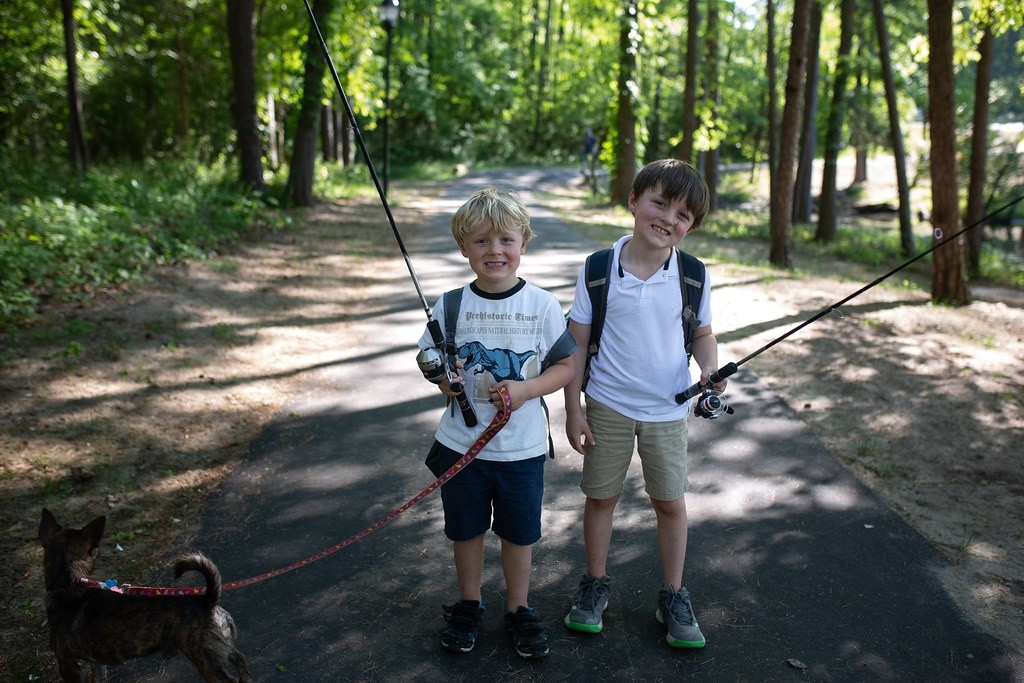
[563,158,729,648]
[579,130,600,176]
[415,187,578,659]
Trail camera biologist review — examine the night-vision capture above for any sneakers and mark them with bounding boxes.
[442,591,486,652]
[656,583,706,648]
[504,602,549,658]
[564,572,611,633]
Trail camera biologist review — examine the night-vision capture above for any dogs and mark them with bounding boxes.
[36,506,256,682]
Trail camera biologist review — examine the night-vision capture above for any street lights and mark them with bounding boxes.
[378,0,401,201]
